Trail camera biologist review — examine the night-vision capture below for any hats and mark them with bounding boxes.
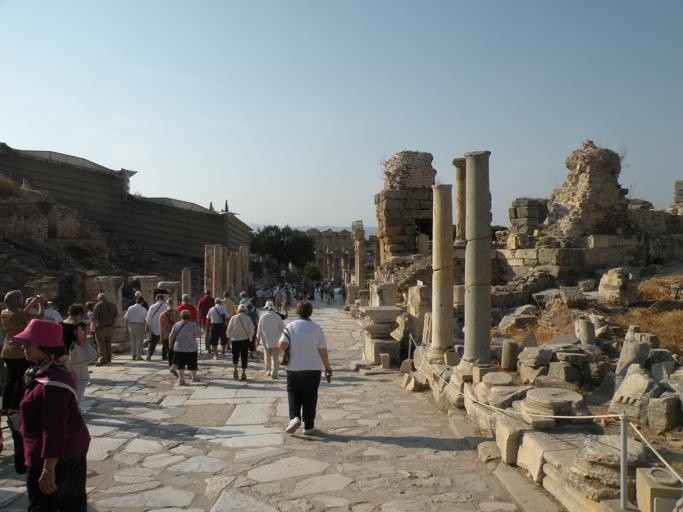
[12,319,66,348]
[264,301,275,311]
[181,310,192,316]
[215,297,225,303]
[237,304,248,312]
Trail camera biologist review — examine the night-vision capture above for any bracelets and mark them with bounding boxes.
[43,468,55,473]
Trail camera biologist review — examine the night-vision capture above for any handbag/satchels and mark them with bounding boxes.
[249,336,255,351]
[278,344,290,365]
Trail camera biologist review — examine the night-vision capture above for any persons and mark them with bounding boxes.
[199,289,291,363]
[125,290,149,360]
[13,318,92,511]
[145,294,202,385]
[92,293,118,368]
[226,303,255,380]
[0,280,98,474]
[277,300,332,434]
[257,301,285,380]
[247,280,337,300]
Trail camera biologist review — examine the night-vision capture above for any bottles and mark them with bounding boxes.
[326,372,332,385]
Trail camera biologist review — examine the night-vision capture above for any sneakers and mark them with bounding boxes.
[303,425,319,434]
[286,417,302,433]
[178,379,186,385]
[192,376,201,382]
[170,367,179,377]
